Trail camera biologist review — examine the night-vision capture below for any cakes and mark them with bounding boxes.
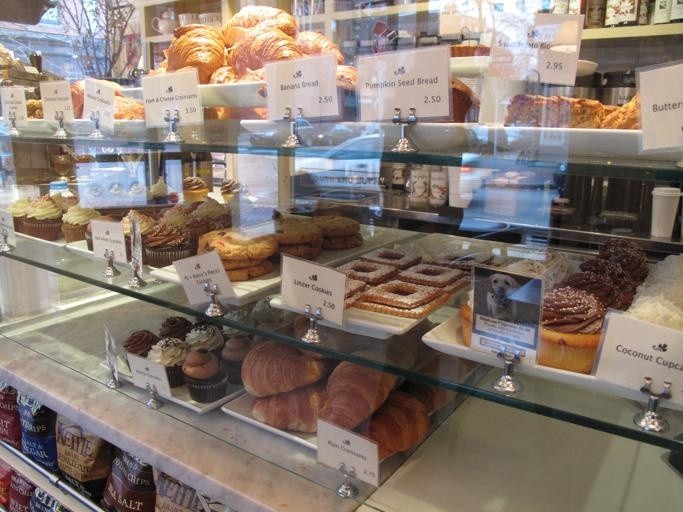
[459,237,683,376]
[122,294,305,400]
[5,176,251,268]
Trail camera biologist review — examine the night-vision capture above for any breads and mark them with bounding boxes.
[148,5,360,118]
[240,341,480,464]
[22,78,144,119]
[450,44,643,133]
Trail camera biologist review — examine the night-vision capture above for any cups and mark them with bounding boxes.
[391,162,473,208]
[650,186,682,239]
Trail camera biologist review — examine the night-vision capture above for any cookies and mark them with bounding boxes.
[194,216,362,281]
[314,245,494,317]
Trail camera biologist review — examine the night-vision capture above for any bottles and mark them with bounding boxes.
[553,0,683,29]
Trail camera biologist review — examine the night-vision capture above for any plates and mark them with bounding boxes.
[61,118,97,138]
[423,246,683,413]
[221,391,462,466]
[66,238,159,274]
[175,119,290,147]
[150,224,421,306]
[99,119,171,142]
[408,122,484,157]
[113,362,244,414]
[489,127,644,159]
[271,233,506,344]
[293,120,405,154]
[17,117,57,138]
